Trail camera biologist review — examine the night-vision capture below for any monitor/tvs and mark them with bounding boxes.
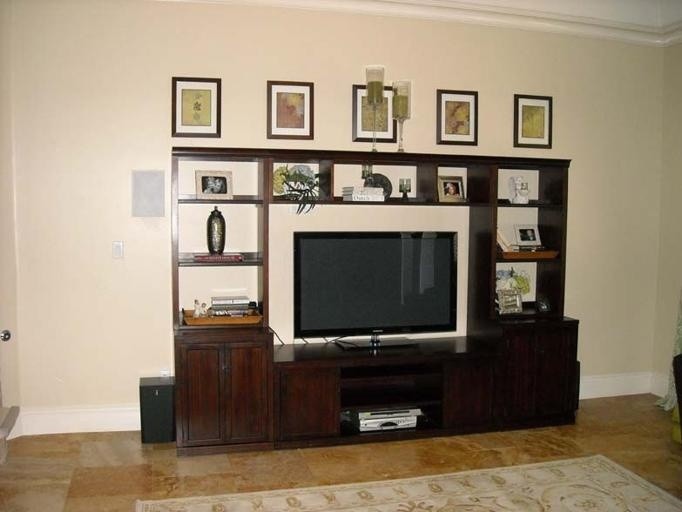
[293,231,457,351]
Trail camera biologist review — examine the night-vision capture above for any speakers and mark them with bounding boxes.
[140,377,176,443]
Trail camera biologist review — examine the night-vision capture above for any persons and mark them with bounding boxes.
[522,229,535,241]
[444,183,460,197]
[210,178,226,194]
[204,177,216,193]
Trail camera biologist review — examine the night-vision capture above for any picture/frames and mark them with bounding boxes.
[172,77,221,138]
[436,89,478,145]
[514,224,542,246]
[195,170,232,200]
[353,84,397,143]
[498,288,522,315]
[267,80,314,140]
[514,94,552,149]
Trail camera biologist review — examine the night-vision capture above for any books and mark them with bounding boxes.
[342,186,386,202]
[207,296,257,318]
[194,254,244,262]
[503,246,545,252]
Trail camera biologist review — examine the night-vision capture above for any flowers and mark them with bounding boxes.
[499,268,530,295]
[273,166,325,209]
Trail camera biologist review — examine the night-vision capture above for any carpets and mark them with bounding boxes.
[135,454,682,512]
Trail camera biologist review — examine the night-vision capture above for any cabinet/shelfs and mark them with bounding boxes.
[173,156,270,333]
[466,159,569,321]
[278,367,337,436]
[481,324,571,430]
[272,156,484,208]
[177,334,270,449]
[341,352,445,439]
